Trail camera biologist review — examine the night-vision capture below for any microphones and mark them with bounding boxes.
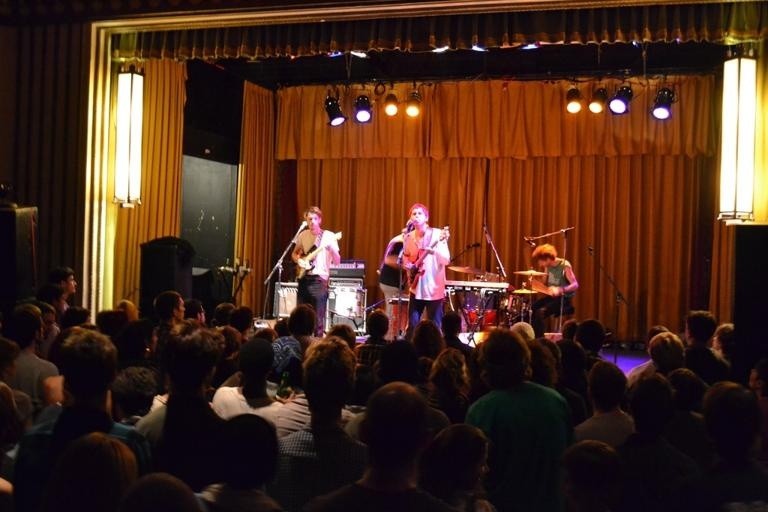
[408,218,419,228]
[300,221,307,230]
[523,236,536,248]
[589,247,594,255]
[482,224,492,244]
[467,243,480,249]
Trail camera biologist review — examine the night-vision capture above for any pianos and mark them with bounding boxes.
[445,280,509,295]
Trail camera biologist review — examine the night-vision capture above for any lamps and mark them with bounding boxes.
[715,41,759,222]
[323,81,425,126]
[114,63,144,209]
[566,76,674,120]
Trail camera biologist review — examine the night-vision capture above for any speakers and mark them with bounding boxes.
[140,238,191,320]
[730,224,768,380]
[0,202,39,301]
[192,268,233,317]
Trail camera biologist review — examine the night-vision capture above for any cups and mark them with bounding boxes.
[544,331,563,345]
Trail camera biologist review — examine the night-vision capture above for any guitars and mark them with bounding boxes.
[287,231,342,281]
[407,225,449,289]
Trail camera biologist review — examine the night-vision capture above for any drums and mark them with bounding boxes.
[467,307,482,324]
[498,293,530,315]
[483,309,497,326]
[389,298,410,341]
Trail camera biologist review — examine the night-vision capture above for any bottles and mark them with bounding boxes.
[276,371,294,399]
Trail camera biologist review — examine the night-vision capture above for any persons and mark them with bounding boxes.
[523,243,580,337]
[401,202,450,340]
[291,205,341,336]
[0,264,768,512]
[378,226,406,341]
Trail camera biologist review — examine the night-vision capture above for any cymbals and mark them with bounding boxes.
[447,266,484,274]
[475,271,504,282]
[510,289,536,294]
[512,270,546,276]
